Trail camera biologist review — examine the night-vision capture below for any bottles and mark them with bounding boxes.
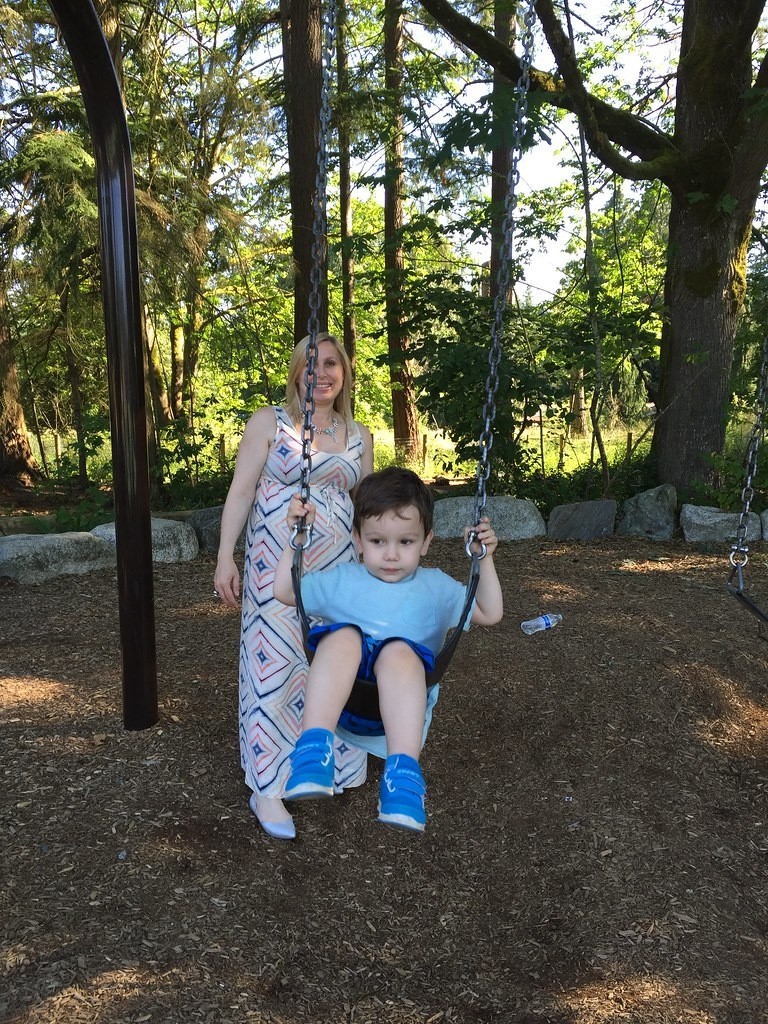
[520,613,562,636]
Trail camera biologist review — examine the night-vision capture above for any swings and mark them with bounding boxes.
[289,0,538,719]
[723,342,768,630]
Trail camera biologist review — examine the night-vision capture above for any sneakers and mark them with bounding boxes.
[288,726,338,793]
[378,753,429,828]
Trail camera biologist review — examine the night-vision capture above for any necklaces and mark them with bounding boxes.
[312,418,339,444]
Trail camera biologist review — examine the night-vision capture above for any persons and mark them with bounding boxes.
[273,467,503,832]
[215,334,374,839]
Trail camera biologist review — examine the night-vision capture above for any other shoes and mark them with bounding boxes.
[248,792,299,840]
